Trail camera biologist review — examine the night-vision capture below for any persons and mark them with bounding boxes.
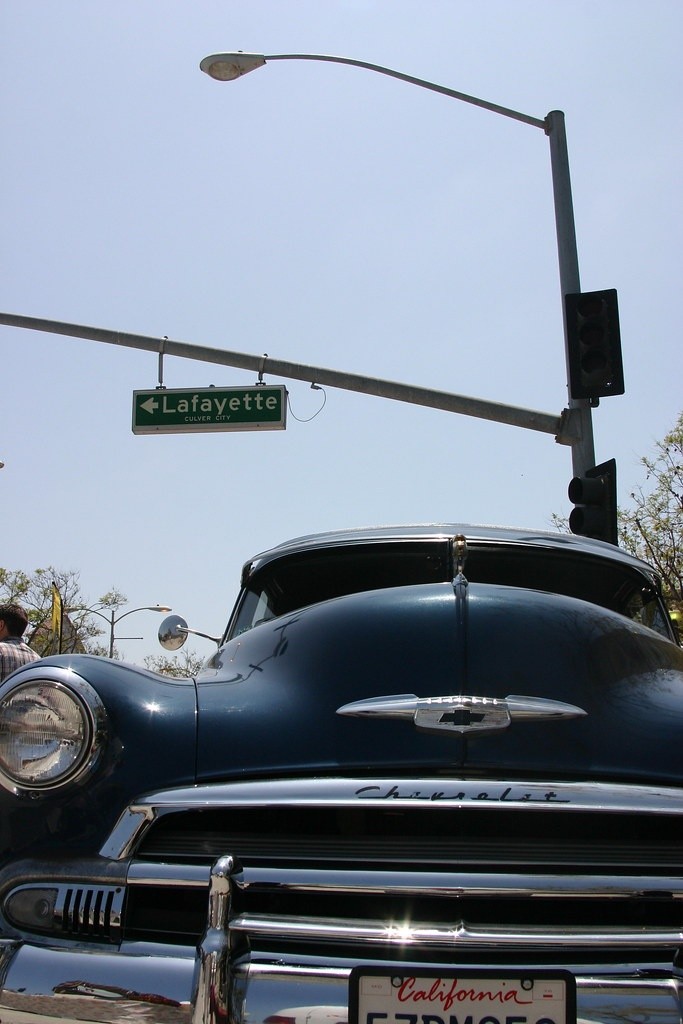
[0,603,42,686]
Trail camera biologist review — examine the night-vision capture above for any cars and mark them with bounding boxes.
[0,522,683,1024]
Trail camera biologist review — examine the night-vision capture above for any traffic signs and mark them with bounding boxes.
[132,384,287,435]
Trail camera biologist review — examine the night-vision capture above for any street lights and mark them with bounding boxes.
[201,48,595,483]
[62,604,172,661]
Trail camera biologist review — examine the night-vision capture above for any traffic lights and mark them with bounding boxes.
[569,458,618,547]
[668,608,683,628]
